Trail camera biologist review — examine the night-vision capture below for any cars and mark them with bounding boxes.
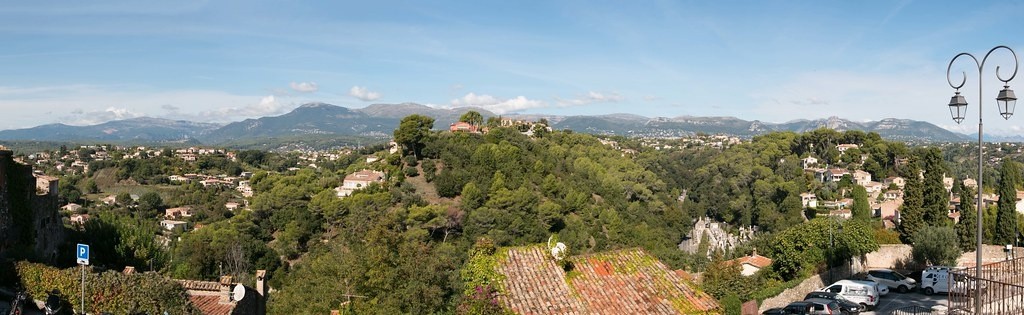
[849,280,890,297]
[868,268,919,294]
[803,299,843,315]
[804,290,861,315]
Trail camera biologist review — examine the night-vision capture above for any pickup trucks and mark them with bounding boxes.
[762,301,823,315]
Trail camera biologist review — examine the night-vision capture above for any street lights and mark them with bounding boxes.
[947,45,1018,314]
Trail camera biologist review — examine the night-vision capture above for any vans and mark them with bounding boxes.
[921,265,989,297]
[815,279,881,312]
